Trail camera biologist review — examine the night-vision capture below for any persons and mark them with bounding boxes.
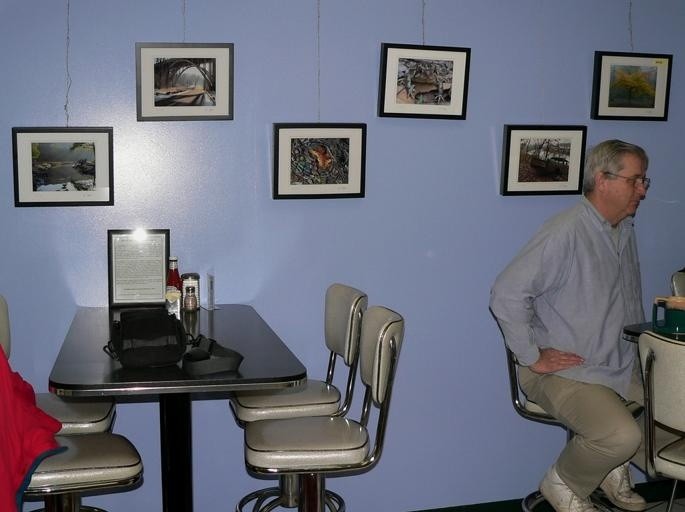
[488,140,651,512]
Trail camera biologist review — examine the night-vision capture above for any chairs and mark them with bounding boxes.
[237,306,404,512]
[672,268,685,296]
[0,343,144,512]
[230,283,369,512]
[488,304,645,512]
[637,329,685,511]
[0,296,116,435]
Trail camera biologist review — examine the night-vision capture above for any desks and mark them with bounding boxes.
[623,316,665,344]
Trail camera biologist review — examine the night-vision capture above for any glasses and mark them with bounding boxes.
[603,171,651,190]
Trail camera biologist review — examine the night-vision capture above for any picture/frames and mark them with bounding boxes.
[107,228,171,309]
[590,50,673,121]
[377,42,472,121]
[500,124,588,196]
[272,122,367,200]
[12,126,114,208]
[135,42,234,121]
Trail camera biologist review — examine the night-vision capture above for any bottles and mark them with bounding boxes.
[167,257,200,319]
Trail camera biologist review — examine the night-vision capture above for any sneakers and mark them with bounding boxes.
[599,459,648,512]
[539,464,599,512]
